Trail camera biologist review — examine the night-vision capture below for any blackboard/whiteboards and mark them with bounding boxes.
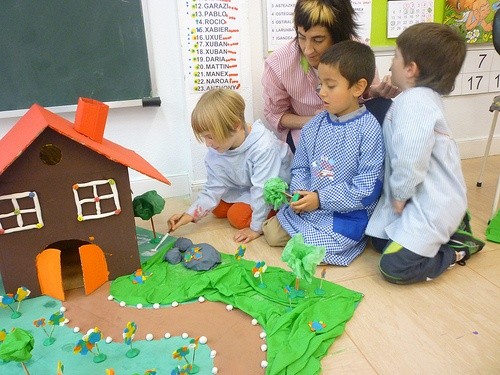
[0,0,161,118]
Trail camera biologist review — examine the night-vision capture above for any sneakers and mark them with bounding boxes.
[459,210,472,234]
[448,229,486,265]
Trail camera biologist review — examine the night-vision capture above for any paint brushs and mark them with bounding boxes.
[153,211,186,251]
